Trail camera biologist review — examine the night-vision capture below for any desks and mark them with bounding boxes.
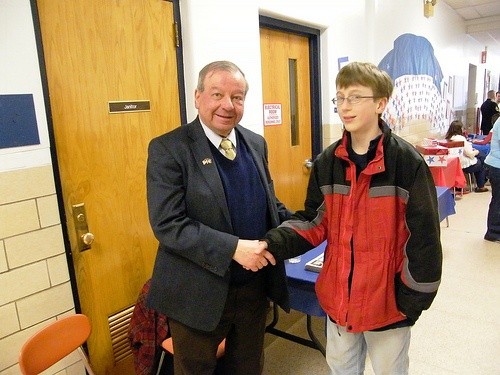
[265,185,449,359]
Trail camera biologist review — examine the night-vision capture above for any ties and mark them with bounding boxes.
[220,139,236,160]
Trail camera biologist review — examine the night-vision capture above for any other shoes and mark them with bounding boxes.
[475,186,487,192]
[485,232,500,241]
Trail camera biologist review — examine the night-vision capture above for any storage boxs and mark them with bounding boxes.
[416,144,448,167]
[422,137,436,147]
[437,139,464,158]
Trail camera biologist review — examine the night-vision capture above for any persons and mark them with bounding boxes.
[145,60,296,375]
[480,90,500,135]
[445,120,489,192]
[483,116,500,242]
[472,113,500,145]
[242,61,443,375]
[494,92,500,112]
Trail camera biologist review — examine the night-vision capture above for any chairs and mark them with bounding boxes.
[156,337,225,375]
[19,314,95,375]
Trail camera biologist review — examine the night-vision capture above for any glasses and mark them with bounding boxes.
[332,95,378,104]
